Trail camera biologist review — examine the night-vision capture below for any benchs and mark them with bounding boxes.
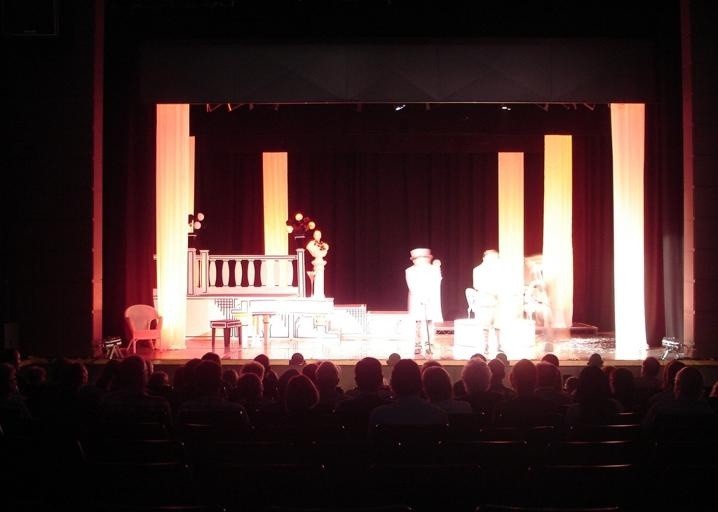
[3,412,716,510]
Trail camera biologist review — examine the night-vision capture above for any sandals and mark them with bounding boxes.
[415,342,422,355]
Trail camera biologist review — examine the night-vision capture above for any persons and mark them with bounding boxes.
[472,250,509,356]
[405,248,445,356]
[1,353,717,512]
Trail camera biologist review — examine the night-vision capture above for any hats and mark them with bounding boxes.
[409,248,433,260]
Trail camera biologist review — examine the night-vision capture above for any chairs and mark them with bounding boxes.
[125,303,163,353]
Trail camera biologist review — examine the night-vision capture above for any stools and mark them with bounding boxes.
[212,320,244,349]
